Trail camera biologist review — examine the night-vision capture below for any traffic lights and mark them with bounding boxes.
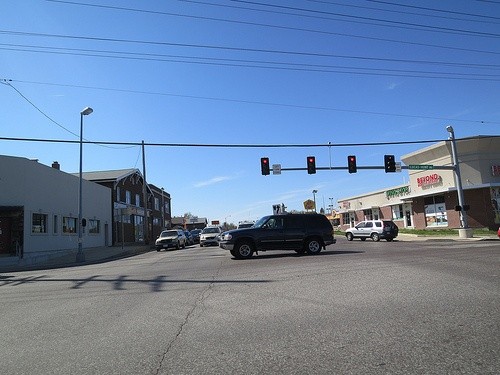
[307,156,316,174]
[347,156,357,173]
[384,154,396,173]
[260,157,270,175]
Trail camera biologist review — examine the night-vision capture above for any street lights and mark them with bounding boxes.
[312,189,319,213]
[75,106,94,263]
[446,125,473,238]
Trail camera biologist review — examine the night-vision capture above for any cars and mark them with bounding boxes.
[155,226,202,251]
[198,226,222,247]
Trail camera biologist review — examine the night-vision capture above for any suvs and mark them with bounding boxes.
[218,214,336,259]
[345,219,398,243]
